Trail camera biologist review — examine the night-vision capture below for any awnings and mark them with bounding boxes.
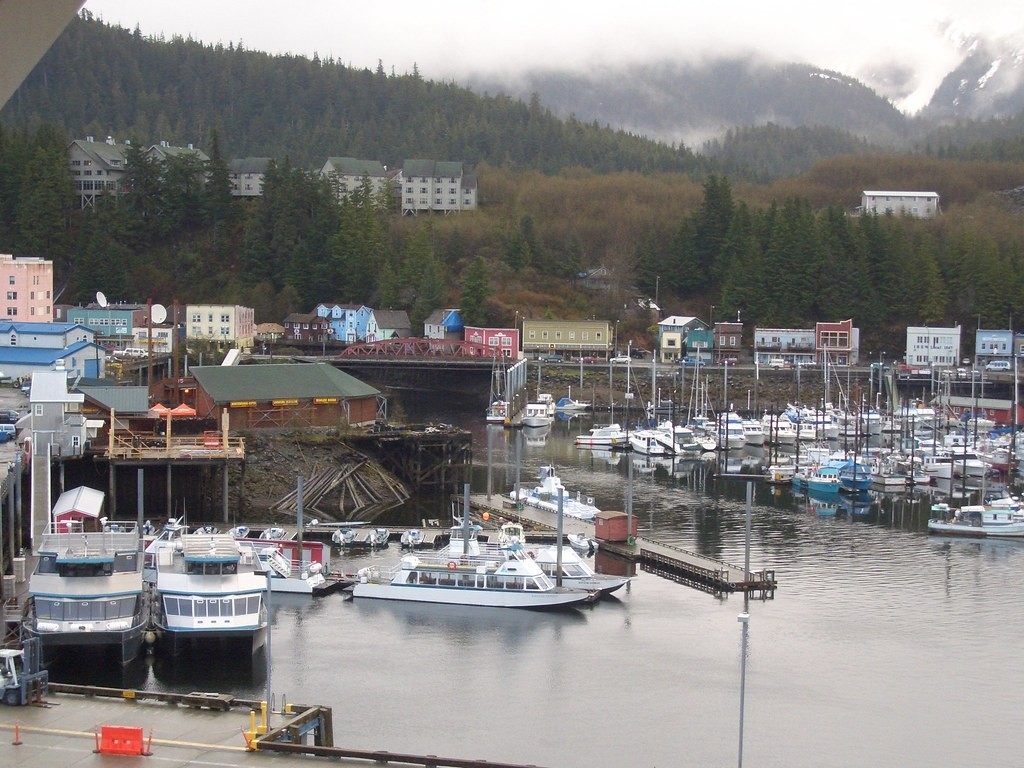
[164,381,197,390]
[977,352,1012,358]
[138,336,165,343]
[109,335,135,339]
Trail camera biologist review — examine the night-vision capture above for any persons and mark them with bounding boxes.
[846,360,874,371]
[671,358,688,367]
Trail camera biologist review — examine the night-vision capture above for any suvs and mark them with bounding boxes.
[609,356,632,364]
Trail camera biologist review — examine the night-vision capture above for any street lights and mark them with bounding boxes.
[656,276,660,304]
[515,311,518,329]
[710,305,714,330]
[615,320,620,358]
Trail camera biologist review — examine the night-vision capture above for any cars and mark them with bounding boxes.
[768,359,784,368]
[873,365,890,372]
[962,357,971,365]
[678,356,706,367]
[956,368,967,380]
[1,408,19,441]
[576,356,597,364]
[798,360,816,366]
[542,354,563,363]
[899,364,922,372]
[716,358,740,366]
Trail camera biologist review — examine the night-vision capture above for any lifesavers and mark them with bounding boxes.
[447,561,457,571]
[587,498,593,503]
[526,550,534,559]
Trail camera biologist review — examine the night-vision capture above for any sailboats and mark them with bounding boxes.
[20,341,1024,658]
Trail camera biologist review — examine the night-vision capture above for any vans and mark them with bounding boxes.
[123,348,149,359]
[985,360,1011,372]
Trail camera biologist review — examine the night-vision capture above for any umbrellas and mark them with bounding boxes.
[149,400,196,417]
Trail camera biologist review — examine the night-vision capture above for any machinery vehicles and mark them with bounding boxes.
[0,620,61,710]
